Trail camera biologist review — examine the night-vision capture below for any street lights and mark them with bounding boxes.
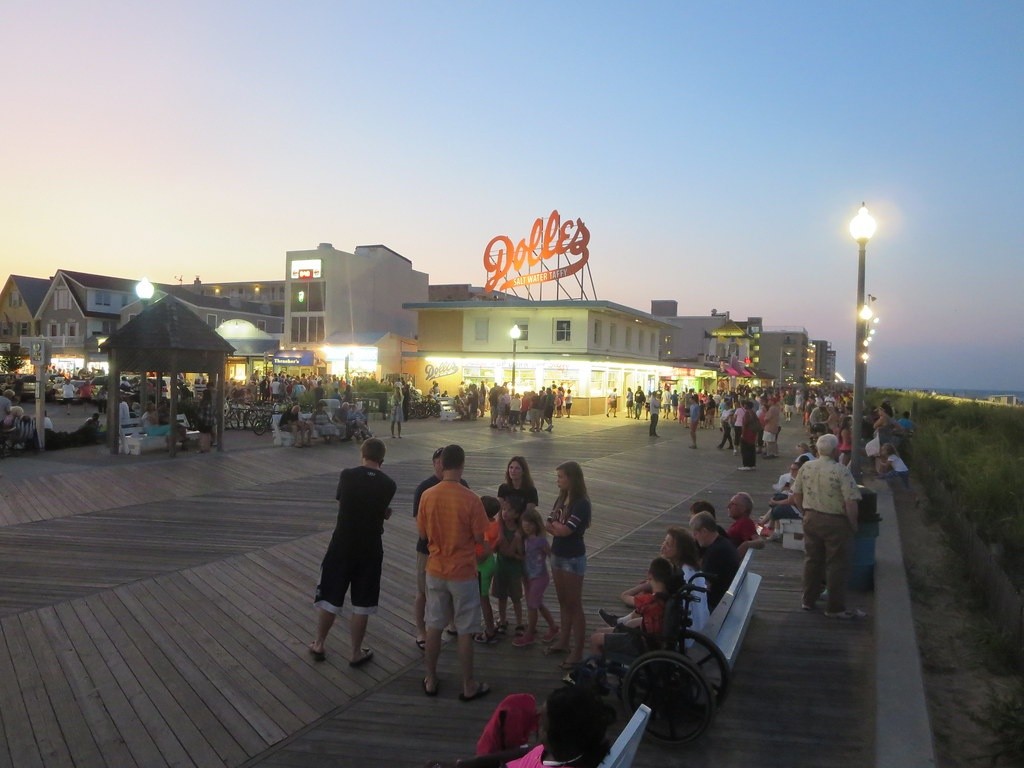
[136,274,155,427]
[510,325,522,402]
[858,303,878,414]
[848,200,878,482]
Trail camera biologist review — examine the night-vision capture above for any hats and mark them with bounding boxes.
[433,447,445,460]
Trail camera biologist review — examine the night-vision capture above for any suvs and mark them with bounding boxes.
[88,375,108,404]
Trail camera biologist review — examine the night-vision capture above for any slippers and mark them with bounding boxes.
[309,641,325,662]
[423,676,438,696]
[416,639,425,650]
[459,681,491,700]
[349,647,373,668]
[560,660,584,669]
[447,629,458,636]
[544,642,568,655]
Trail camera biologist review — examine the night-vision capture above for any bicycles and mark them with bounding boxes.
[405,393,441,419]
[222,396,314,436]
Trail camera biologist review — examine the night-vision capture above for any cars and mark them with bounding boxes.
[119,375,196,407]
[54,379,90,404]
[20,374,53,401]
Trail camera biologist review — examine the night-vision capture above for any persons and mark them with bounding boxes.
[86,412,101,431]
[413,444,591,702]
[424,687,617,768]
[250,371,371,448]
[430,379,572,432]
[352,372,412,438]
[591,493,765,693]
[119,372,252,453]
[44,365,107,414]
[312,438,398,667]
[794,434,860,619]
[0,389,52,448]
[606,385,913,540]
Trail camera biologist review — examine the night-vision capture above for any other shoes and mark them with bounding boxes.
[737,465,756,471]
[825,608,867,620]
[822,589,830,596]
[802,602,812,609]
[766,534,781,542]
[598,608,619,628]
[690,445,697,449]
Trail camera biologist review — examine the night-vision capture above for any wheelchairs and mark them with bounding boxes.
[561,572,732,751]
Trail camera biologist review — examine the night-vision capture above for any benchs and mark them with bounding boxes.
[687,547,763,694]
[272,414,337,446]
[119,413,201,454]
[437,397,460,422]
[597,702,652,768]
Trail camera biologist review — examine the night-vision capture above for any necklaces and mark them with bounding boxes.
[443,479,459,482]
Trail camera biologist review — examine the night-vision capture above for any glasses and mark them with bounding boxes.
[728,502,742,506]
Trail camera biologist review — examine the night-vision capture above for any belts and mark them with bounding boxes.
[805,509,844,516]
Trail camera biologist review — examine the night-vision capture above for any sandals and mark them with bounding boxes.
[512,632,535,647]
[472,629,498,643]
[496,620,508,634]
[515,625,525,636]
[542,626,560,642]
